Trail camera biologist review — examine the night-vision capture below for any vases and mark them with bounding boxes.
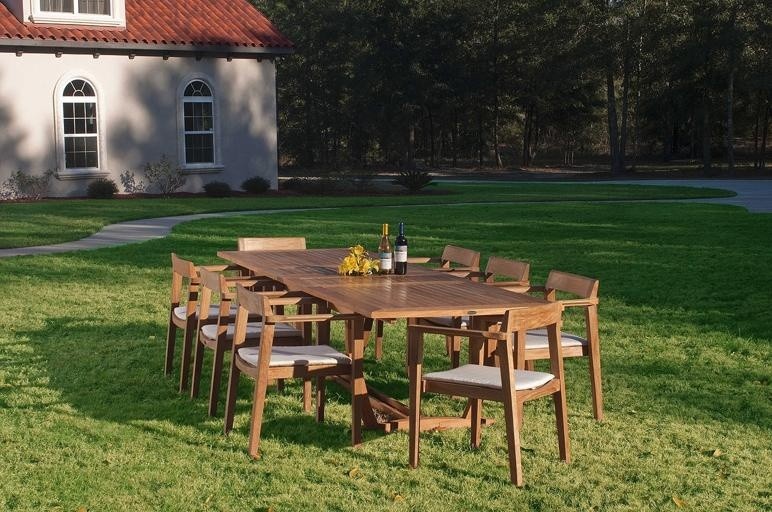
[351,269,374,275]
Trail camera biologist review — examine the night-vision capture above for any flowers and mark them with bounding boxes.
[336,245,380,276]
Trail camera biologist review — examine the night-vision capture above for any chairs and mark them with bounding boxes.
[407,301,572,485]
[239,236,307,315]
[164,253,262,393]
[225,282,364,455]
[190,268,313,415]
[511,270,604,419]
[417,255,529,366]
[376,245,480,358]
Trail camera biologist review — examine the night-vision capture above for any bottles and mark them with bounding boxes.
[394,222,409,275]
[378,224,393,274]
